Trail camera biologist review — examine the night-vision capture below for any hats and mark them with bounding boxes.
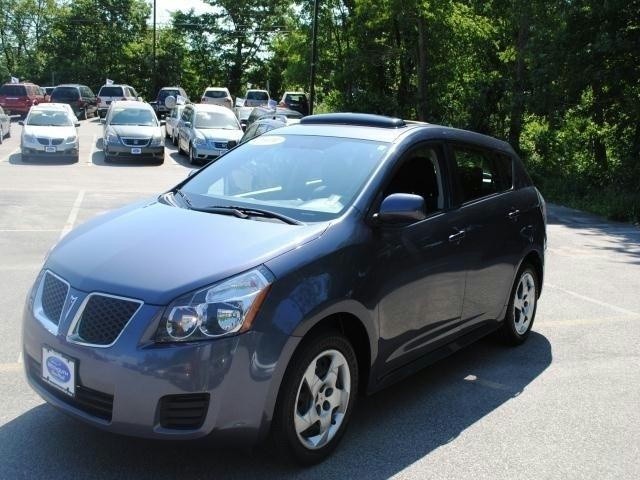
[19,107,549,465]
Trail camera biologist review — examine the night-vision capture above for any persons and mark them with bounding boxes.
[350,146,400,206]
